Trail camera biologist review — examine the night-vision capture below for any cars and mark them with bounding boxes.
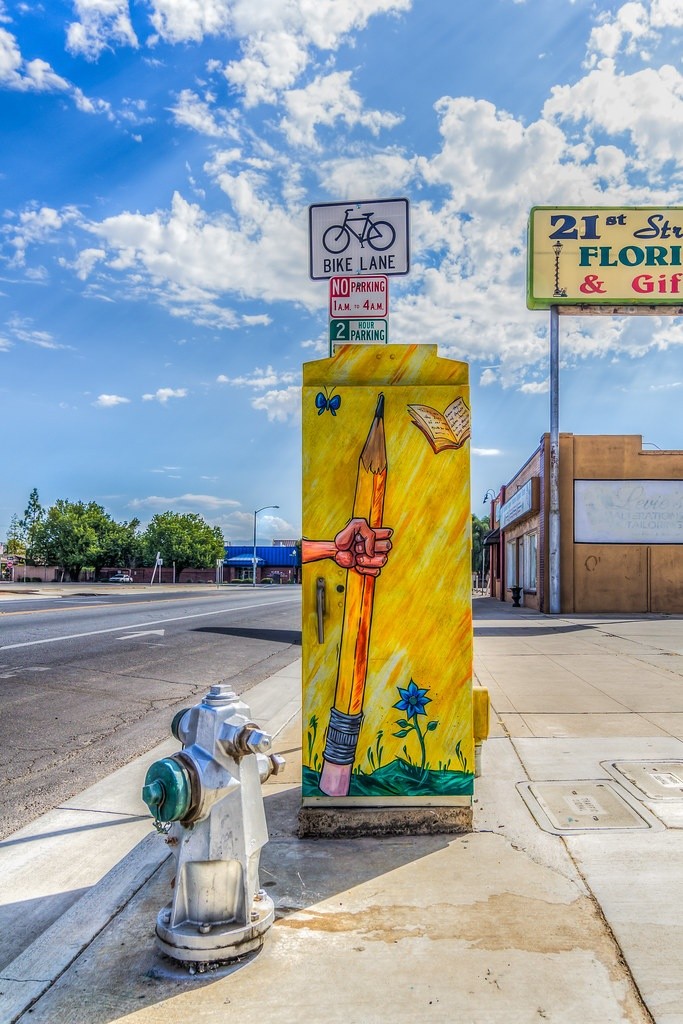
[110,575,133,583]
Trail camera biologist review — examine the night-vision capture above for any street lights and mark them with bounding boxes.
[251,506,280,588]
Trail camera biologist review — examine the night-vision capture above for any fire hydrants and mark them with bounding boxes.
[138,684,286,978]
